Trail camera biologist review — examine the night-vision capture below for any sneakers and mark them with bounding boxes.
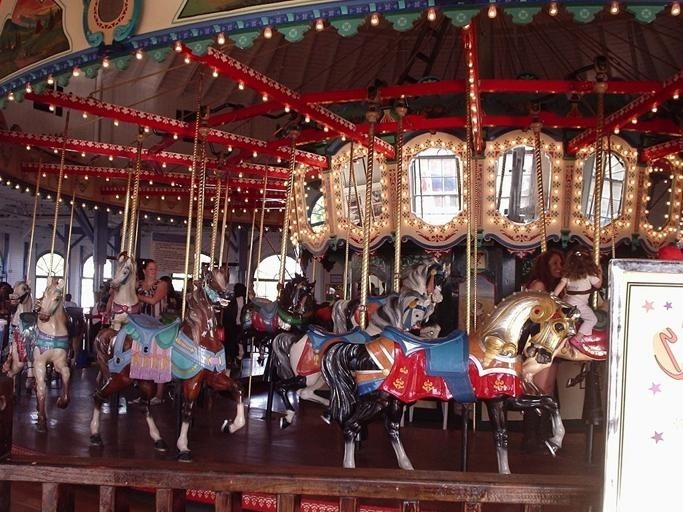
[571,336,581,347]
[134,396,165,404]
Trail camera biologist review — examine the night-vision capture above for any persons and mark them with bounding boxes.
[92,259,182,406]
[426,264,444,293]
[554,244,604,350]
[64,294,77,312]
[520,249,561,443]
[223,283,245,370]
[0,282,13,318]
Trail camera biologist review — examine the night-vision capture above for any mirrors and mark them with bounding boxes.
[567,134,639,256]
[330,141,394,258]
[480,129,564,260]
[292,162,330,262]
[636,151,683,256]
[396,130,478,258]
